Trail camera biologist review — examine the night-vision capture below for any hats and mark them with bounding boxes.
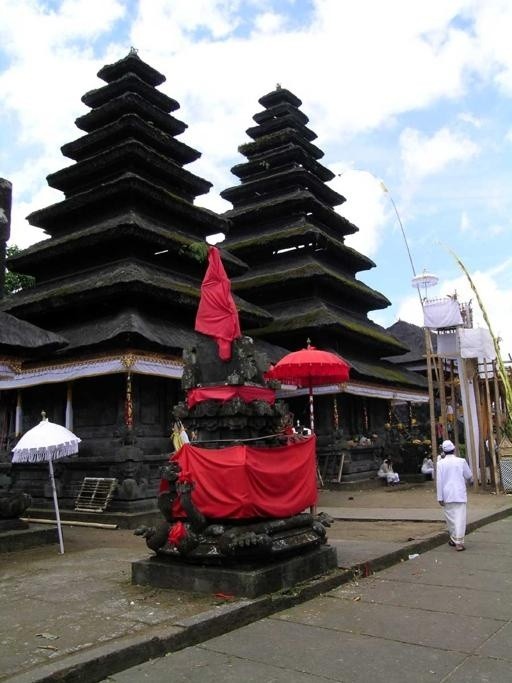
[441,439,455,452]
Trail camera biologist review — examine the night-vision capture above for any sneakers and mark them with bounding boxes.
[448,539,465,551]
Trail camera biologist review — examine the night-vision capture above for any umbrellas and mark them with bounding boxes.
[11,411,84,555]
[407,266,442,299]
[265,338,352,434]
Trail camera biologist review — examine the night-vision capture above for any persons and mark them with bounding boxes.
[383,452,401,485]
[419,451,446,481]
[434,438,475,551]
[377,458,395,487]
